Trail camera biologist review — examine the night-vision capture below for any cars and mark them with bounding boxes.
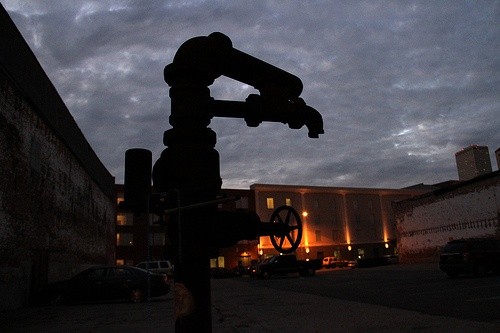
[33,263,172,306]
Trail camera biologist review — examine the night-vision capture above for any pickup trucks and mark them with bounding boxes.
[256,254,322,285]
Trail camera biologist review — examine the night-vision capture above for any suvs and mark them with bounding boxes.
[439,237,499,276]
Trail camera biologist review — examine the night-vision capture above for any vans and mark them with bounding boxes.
[130,261,171,277]
[322,256,341,269]
